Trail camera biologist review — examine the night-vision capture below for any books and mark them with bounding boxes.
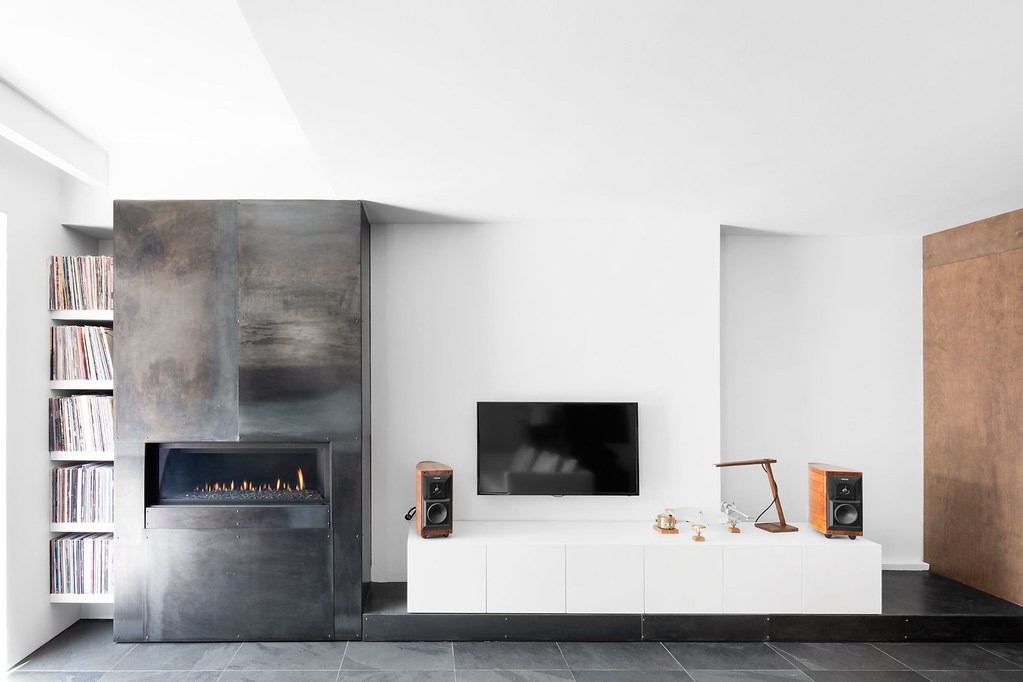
[46,255,113,310]
[48,324,113,380]
[49,532,114,594]
[47,395,115,453]
[51,463,115,522]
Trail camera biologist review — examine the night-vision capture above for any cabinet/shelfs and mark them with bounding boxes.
[48,309,112,606]
[112,200,371,444]
[405,521,884,615]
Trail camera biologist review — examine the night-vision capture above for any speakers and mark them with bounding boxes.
[416,461,454,539]
[808,463,863,540]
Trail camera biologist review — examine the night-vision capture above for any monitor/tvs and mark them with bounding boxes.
[476,401,640,496]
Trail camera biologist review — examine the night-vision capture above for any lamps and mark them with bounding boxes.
[711,457,798,534]
